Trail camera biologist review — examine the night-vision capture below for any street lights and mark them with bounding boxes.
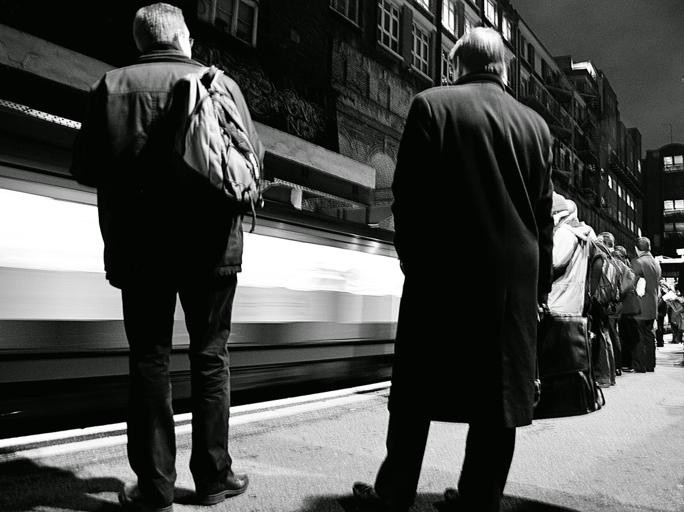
[534,303,606,421]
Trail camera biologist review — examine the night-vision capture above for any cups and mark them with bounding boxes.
[185,38,194,47]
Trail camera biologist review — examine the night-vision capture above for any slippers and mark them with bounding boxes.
[591,241,638,307]
[181,64,265,212]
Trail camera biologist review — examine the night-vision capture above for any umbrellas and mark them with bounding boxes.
[197,468,249,505]
[352,482,387,509]
[117,484,175,512]
[444,487,461,512]
[598,363,659,388]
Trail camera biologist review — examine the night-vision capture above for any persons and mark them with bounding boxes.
[353,26,552,512]
[552,192,661,389]
[71,3,265,512]
[656,283,683,347]
